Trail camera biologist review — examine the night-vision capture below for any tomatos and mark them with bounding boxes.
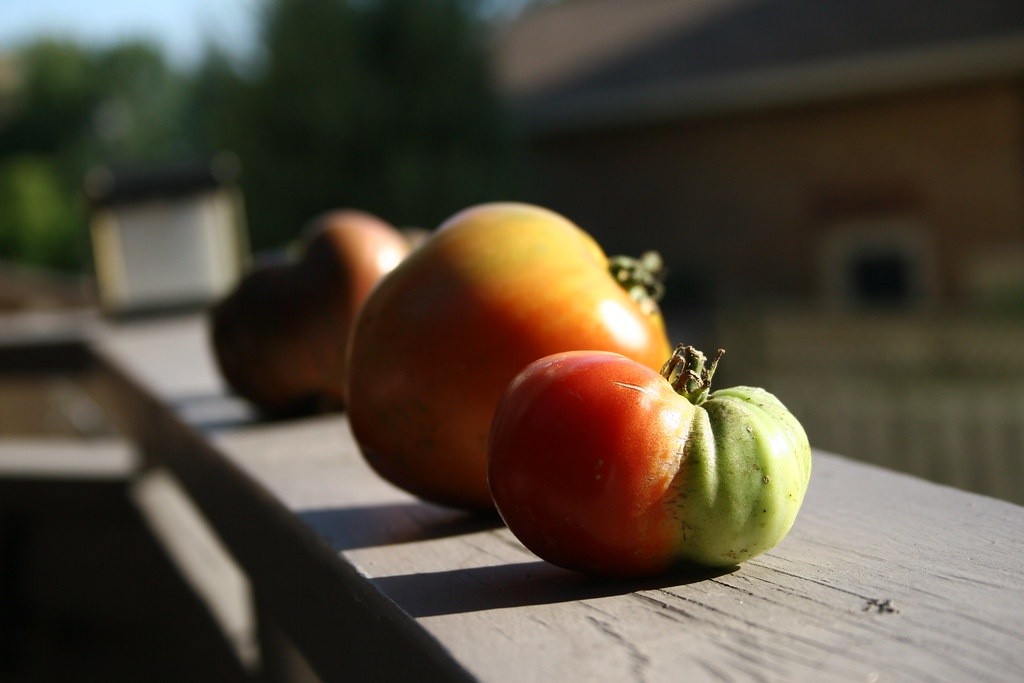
[486,351,811,578]
[209,203,678,514]
